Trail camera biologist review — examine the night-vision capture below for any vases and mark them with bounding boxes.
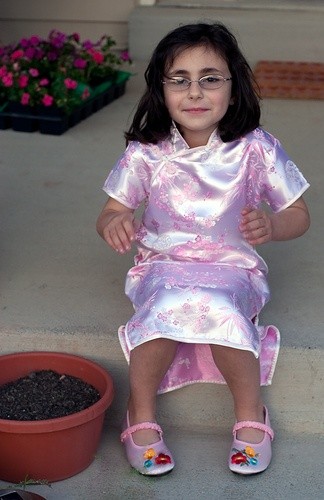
[0,352,114,483]
[0,68,129,135]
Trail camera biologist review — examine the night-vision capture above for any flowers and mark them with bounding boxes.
[0,30,131,109]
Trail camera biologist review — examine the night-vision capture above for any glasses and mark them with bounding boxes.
[160,74,231,90]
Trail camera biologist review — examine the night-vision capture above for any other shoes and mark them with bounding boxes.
[227,405,274,475]
[120,409,176,476]
